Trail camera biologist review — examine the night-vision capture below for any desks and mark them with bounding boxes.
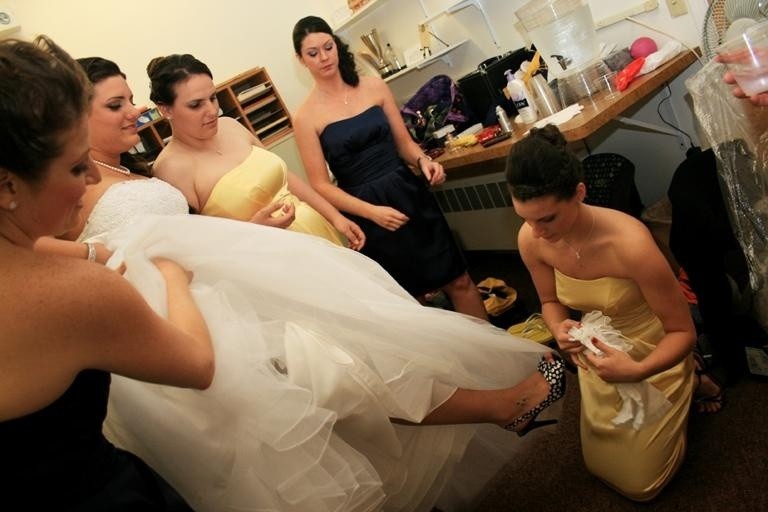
[428,44,703,175]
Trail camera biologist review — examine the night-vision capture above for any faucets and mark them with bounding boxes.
[551,54,570,70]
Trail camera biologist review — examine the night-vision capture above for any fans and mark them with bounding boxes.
[701,0,768,64]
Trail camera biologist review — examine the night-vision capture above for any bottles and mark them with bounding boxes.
[494,105,515,135]
[382,41,407,74]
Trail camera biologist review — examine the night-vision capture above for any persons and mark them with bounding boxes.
[145,52,368,253]
[0,33,218,510]
[714,47,768,108]
[33,54,568,511]
[290,14,492,321]
[505,123,727,505]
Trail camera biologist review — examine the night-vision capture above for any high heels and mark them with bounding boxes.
[502,349,567,437]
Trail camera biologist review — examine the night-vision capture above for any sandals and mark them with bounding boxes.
[692,347,724,414]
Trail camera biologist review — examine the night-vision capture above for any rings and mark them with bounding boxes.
[443,173,447,177]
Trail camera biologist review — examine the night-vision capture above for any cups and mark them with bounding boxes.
[514,1,603,99]
[590,71,622,101]
[528,73,562,118]
[714,19,768,99]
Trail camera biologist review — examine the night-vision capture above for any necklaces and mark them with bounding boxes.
[563,203,596,262]
[216,149,225,155]
[89,160,132,177]
[317,82,351,106]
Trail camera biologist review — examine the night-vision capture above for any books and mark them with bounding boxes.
[136,108,162,130]
[235,81,292,145]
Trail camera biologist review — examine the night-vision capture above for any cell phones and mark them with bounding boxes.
[482,131,513,147]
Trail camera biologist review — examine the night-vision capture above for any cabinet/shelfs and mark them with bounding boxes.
[129,66,294,150]
[332,0,469,84]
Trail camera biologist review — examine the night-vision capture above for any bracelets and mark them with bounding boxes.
[417,156,432,171]
[83,243,96,262]
